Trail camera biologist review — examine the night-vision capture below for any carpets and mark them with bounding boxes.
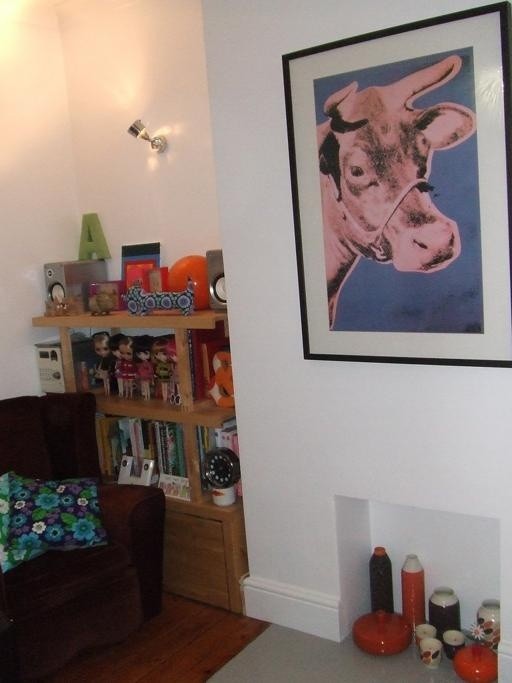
[206,624,498,683]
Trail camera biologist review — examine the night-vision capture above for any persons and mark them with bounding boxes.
[92,331,180,403]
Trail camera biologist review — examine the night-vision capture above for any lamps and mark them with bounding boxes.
[127,120,168,154]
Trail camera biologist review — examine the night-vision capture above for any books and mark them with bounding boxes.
[94,417,242,497]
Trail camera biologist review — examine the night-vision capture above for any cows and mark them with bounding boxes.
[315,55,477,330]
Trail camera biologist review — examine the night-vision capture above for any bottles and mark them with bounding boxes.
[368,546,395,614]
[427,585,462,644]
[476,599,499,655]
[400,553,427,633]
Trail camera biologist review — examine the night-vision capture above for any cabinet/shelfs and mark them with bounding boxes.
[32,308,248,617]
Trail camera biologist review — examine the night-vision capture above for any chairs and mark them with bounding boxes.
[0,392,166,682]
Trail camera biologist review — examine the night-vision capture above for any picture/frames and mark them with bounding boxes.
[281,1,512,368]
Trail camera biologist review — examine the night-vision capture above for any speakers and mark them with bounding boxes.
[41,261,110,315]
[207,247,227,310]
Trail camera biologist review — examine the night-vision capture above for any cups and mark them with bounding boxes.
[442,629,466,660]
[414,623,443,670]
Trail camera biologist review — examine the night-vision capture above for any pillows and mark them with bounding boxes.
[0,466,45,575]
[7,472,108,564]
